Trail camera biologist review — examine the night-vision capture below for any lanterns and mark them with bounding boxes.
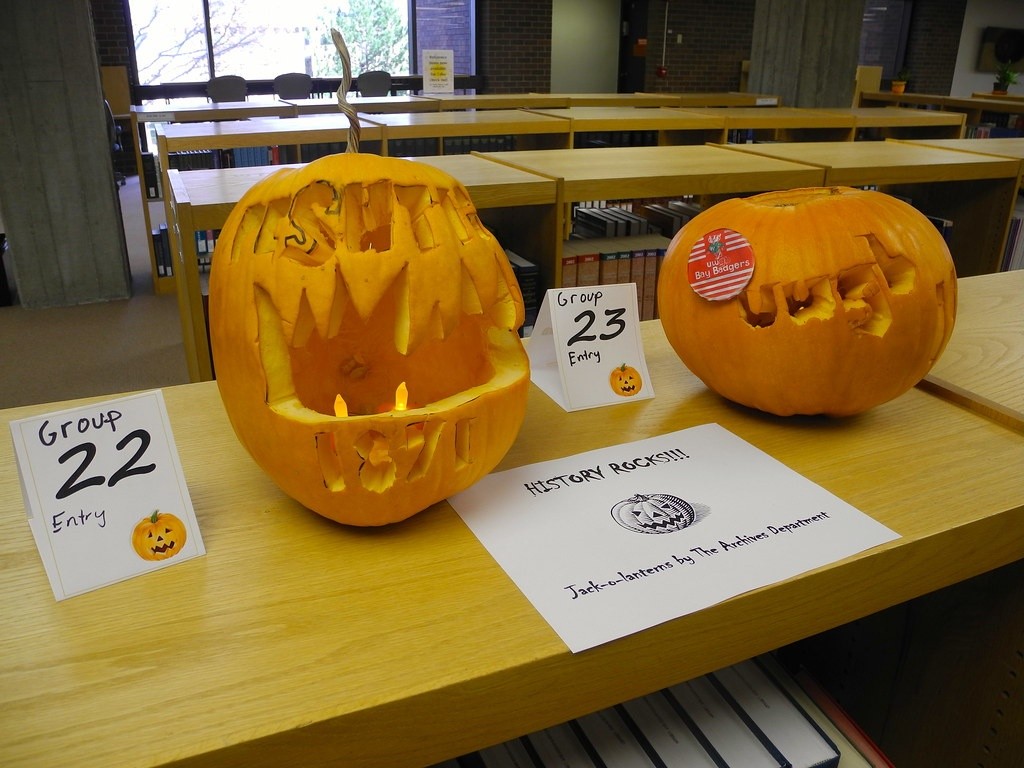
[208,154,529,528]
[657,185,959,418]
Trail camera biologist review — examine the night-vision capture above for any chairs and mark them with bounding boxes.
[105,100,126,190]
[207,75,248,102]
[357,72,392,97]
[274,73,314,99]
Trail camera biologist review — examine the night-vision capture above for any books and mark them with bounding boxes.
[450,647,897,768]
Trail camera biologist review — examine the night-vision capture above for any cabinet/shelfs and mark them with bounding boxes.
[739,61,883,109]
[0,90,1024,768]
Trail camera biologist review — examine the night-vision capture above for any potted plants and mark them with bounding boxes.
[991,60,1022,95]
[891,72,910,94]
